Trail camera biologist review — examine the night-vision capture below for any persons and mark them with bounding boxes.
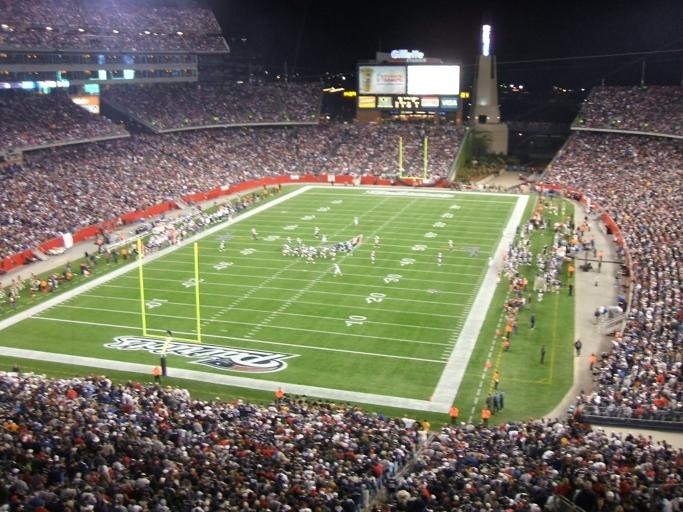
[0,0,682,511]
